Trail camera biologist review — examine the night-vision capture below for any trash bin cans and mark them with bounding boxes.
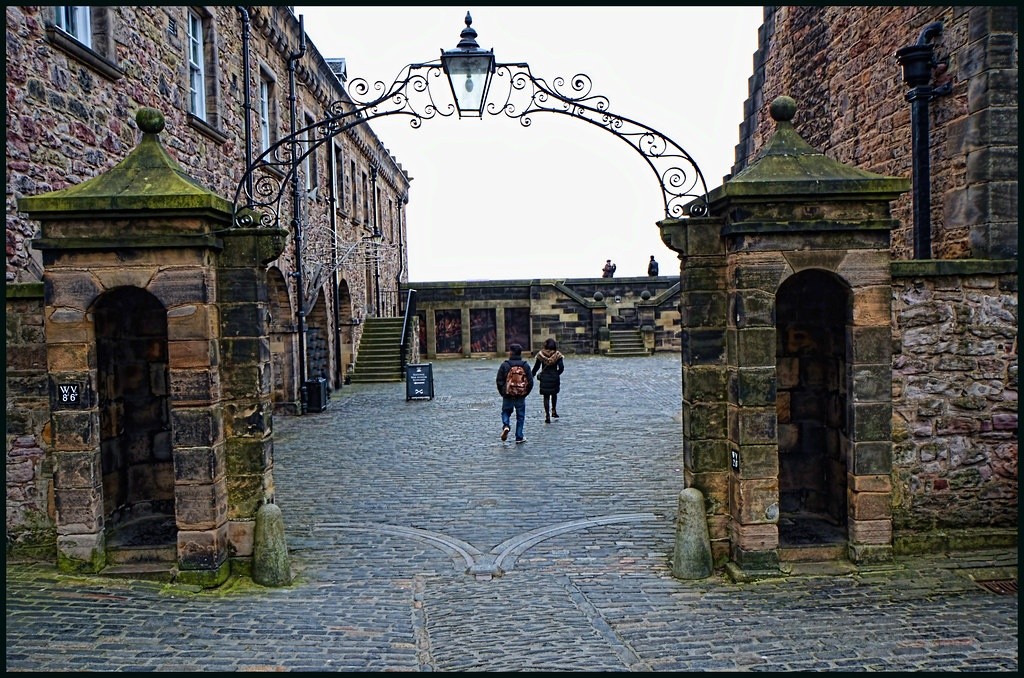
[304,376,327,412]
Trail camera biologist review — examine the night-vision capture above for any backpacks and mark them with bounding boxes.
[505,360,527,396]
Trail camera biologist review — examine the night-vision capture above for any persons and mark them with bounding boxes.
[603,260,616,277]
[648,255,659,276]
[532,338,564,423]
[496,345,534,443]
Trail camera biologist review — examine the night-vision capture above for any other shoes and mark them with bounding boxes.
[501,426,509,441]
[552,413,559,418]
[515,436,526,444]
[545,419,550,423]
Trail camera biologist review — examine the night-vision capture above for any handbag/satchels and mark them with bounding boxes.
[537,372,542,380]
